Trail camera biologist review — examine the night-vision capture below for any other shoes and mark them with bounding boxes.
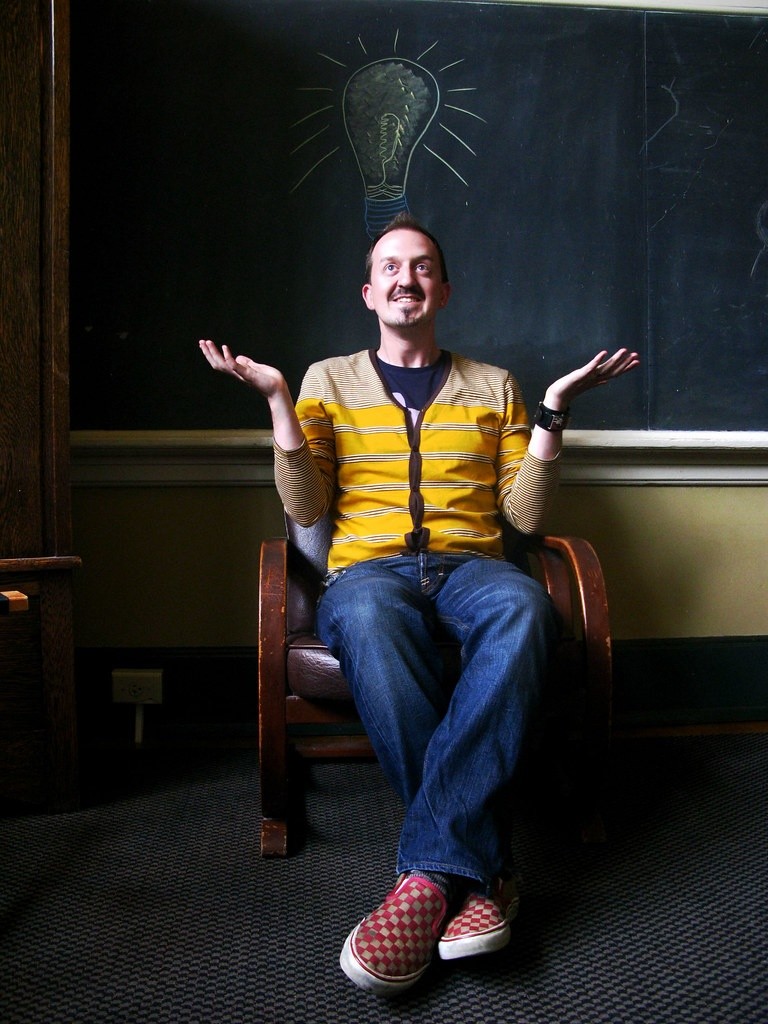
[438,873,520,960]
[339,870,452,996]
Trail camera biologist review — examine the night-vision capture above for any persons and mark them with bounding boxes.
[199,210,640,999]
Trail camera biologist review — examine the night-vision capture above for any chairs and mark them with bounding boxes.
[259,506,612,857]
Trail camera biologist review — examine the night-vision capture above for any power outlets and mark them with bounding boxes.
[111,669,163,703]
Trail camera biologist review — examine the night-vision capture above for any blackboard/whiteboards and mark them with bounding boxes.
[48,0,768,467]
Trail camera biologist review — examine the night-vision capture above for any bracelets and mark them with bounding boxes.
[533,400,573,432]
